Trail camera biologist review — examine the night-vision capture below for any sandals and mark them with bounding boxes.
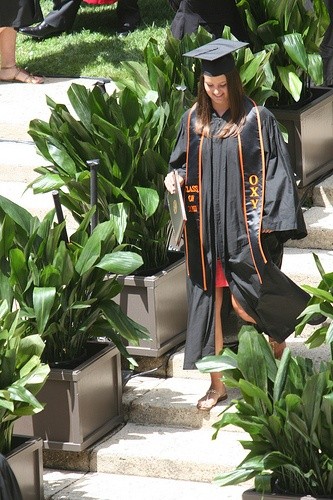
[197,383,228,411]
[0,65,44,84]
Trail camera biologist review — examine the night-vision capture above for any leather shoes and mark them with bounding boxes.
[18,22,70,39]
[116,11,141,35]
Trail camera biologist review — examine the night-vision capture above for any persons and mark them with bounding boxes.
[0,0,244,85]
[165,38,309,407]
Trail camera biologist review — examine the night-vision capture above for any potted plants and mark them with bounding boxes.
[0,0,333,500]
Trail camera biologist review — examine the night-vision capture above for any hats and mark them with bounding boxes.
[182,38,250,76]
